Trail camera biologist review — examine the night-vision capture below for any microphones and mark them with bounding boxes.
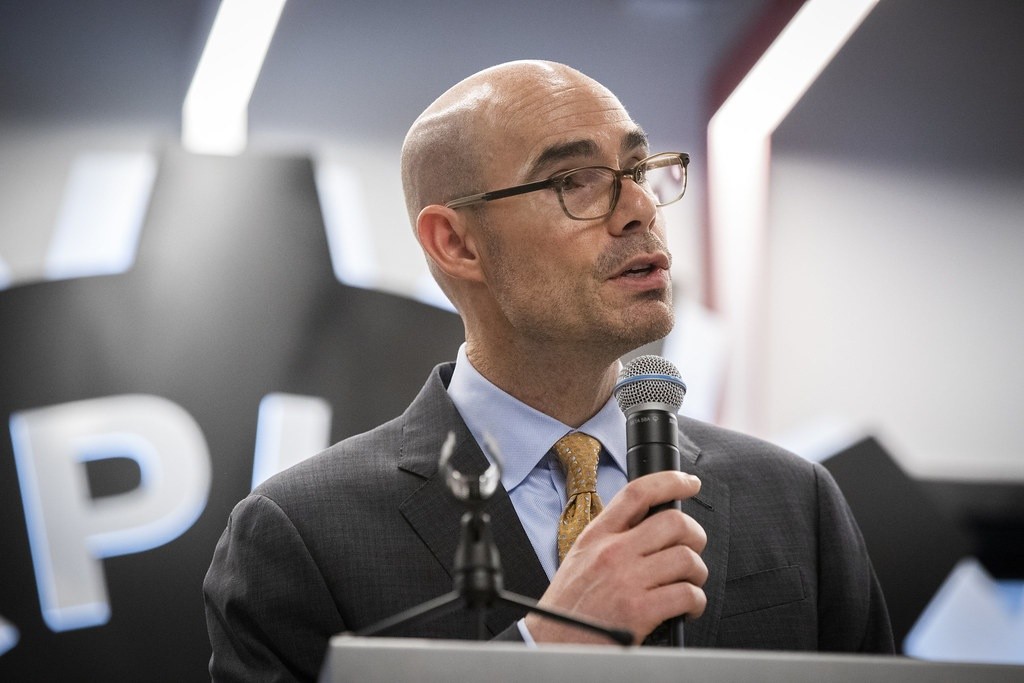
[612,356,687,642]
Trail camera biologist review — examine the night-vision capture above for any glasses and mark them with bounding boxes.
[444,151,690,221]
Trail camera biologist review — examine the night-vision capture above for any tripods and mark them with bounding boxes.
[357,430,637,649]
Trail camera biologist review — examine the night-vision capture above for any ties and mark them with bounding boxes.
[550,433,605,576]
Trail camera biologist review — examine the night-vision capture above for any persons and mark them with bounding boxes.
[201,57,898,683]
